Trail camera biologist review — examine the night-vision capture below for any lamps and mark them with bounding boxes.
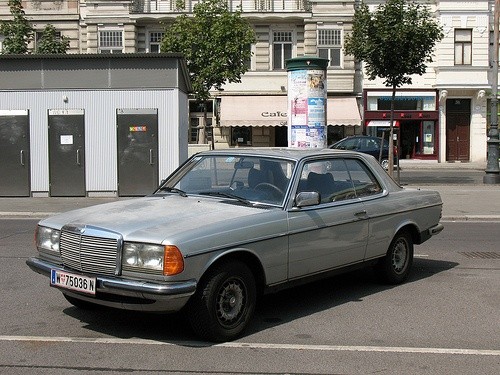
[438,89,449,103]
[477,89,486,102]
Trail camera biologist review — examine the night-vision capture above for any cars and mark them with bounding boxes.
[24,146,445,341]
[327,135,398,171]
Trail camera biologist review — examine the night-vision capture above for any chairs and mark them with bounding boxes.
[298,171,338,204]
[232,165,276,204]
